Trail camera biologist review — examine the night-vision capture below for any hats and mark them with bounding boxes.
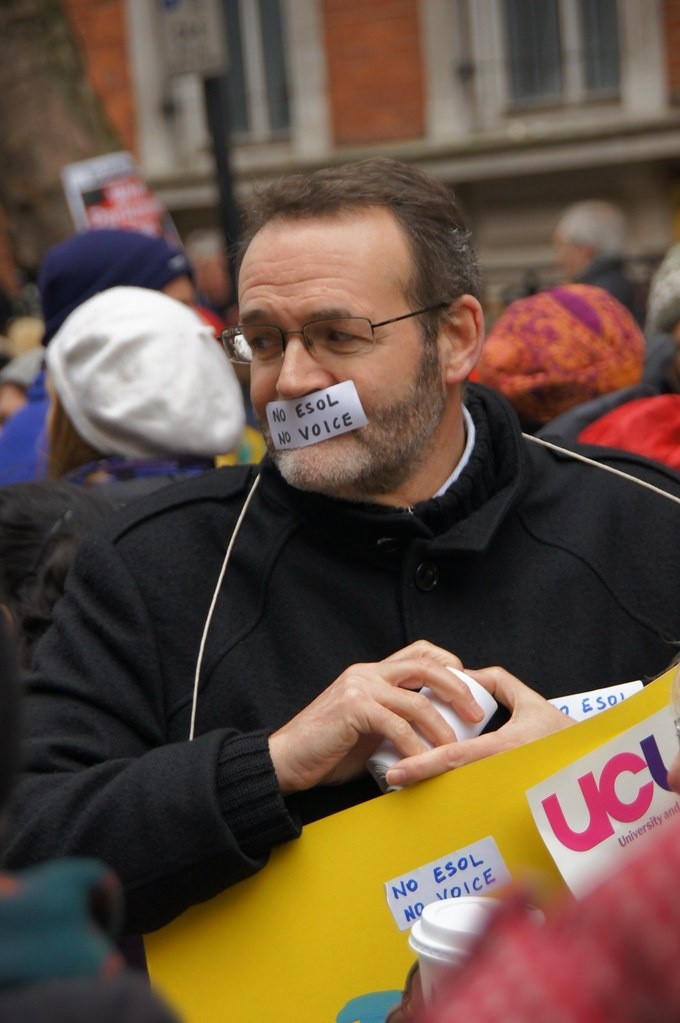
[41,230,195,347]
[45,285,248,463]
[644,243,680,341]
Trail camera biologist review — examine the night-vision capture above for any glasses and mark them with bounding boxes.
[216,303,446,364]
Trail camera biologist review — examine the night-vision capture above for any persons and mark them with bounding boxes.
[478,283,646,423]
[0,155,680,1023]
[0,228,195,484]
[553,201,637,311]
[39,285,247,490]
